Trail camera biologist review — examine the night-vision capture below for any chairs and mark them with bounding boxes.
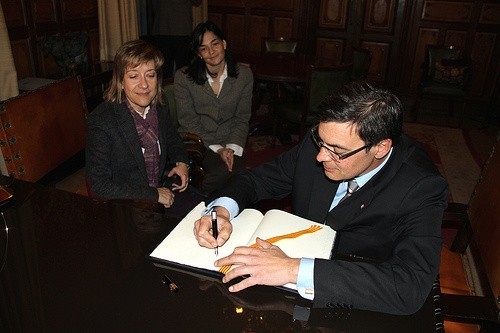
[261,39,353,150]
[410,44,472,127]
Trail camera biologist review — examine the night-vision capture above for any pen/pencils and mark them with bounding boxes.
[211,207,221,256]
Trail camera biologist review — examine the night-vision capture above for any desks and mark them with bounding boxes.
[0,175,436,333]
[16,62,114,177]
[241,54,307,142]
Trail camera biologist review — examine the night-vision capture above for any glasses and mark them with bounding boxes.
[311,124,374,162]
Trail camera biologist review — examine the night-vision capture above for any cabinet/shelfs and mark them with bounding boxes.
[0,0,500,113]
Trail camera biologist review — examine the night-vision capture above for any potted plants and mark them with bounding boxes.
[41,30,89,70]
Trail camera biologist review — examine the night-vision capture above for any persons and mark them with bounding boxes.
[199,278,434,333]
[193,82,449,314]
[175,20,253,193]
[84,40,201,217]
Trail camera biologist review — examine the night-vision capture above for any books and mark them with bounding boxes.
[146,201,336,293]
